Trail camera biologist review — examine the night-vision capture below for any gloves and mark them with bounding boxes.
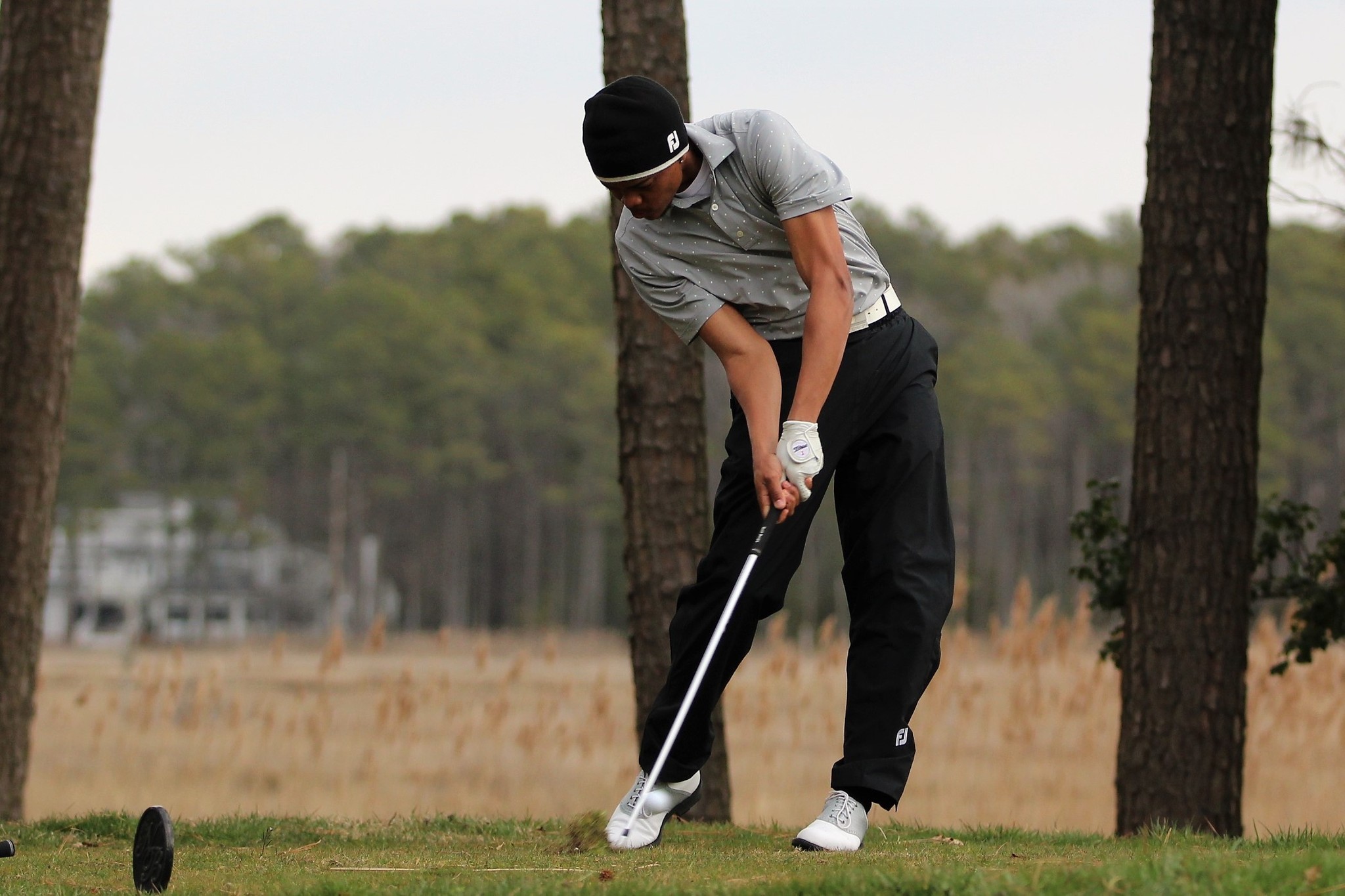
[775,421,824,500]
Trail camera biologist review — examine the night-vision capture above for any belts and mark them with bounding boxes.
[848,282,902,333]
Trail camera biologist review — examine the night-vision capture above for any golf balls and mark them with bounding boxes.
[645,790,672,813]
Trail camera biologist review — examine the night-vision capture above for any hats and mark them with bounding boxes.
[582,75,690,183]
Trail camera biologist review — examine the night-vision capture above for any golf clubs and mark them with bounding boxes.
[603,469,789,856]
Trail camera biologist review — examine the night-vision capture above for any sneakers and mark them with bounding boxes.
[604,766,702,851]
[792,791,869,853]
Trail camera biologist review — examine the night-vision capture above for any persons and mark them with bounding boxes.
[581,75,956,852]
